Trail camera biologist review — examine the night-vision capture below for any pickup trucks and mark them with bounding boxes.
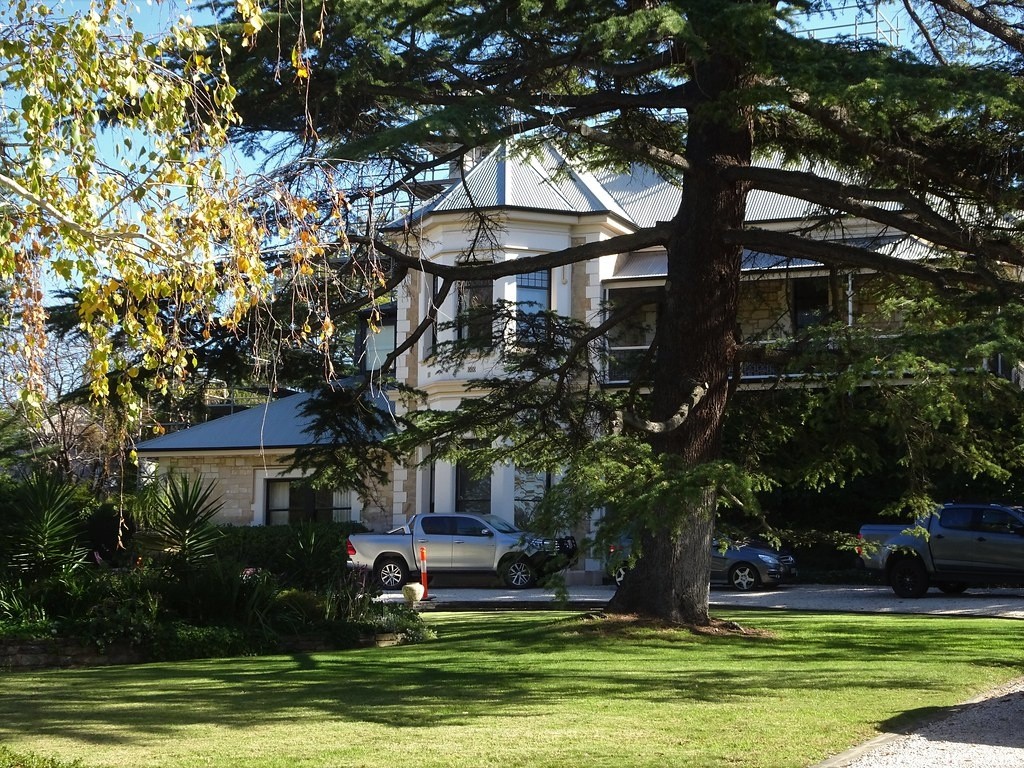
[856,502,1024,600]
[348,513,577,589]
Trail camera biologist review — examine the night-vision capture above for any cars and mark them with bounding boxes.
[609,520,795,590]
